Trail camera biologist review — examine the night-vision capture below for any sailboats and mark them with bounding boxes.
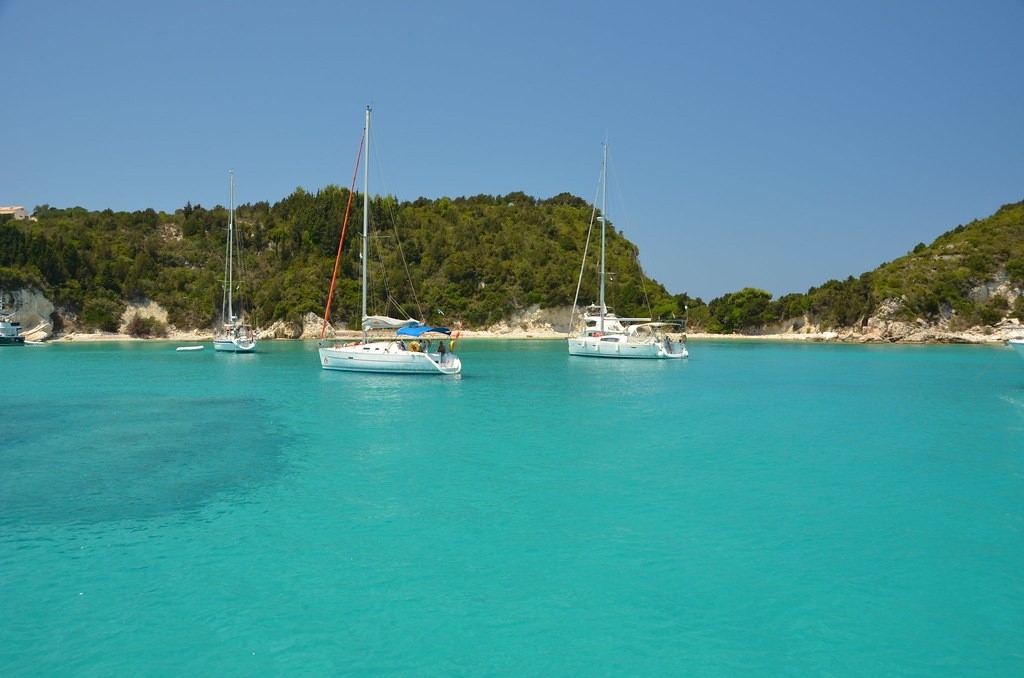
[318,104,462,375]
[568,129,689,360]
[213,168,257,353]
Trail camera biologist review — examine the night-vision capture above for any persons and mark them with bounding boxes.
[399,339,454,363]
[334,341,362,349]
[654,334,687,354]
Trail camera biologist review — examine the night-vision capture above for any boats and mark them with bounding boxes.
[0,312,25,346]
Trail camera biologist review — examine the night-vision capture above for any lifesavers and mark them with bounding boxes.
[450,341,455,352]
[408,340,421,352]
